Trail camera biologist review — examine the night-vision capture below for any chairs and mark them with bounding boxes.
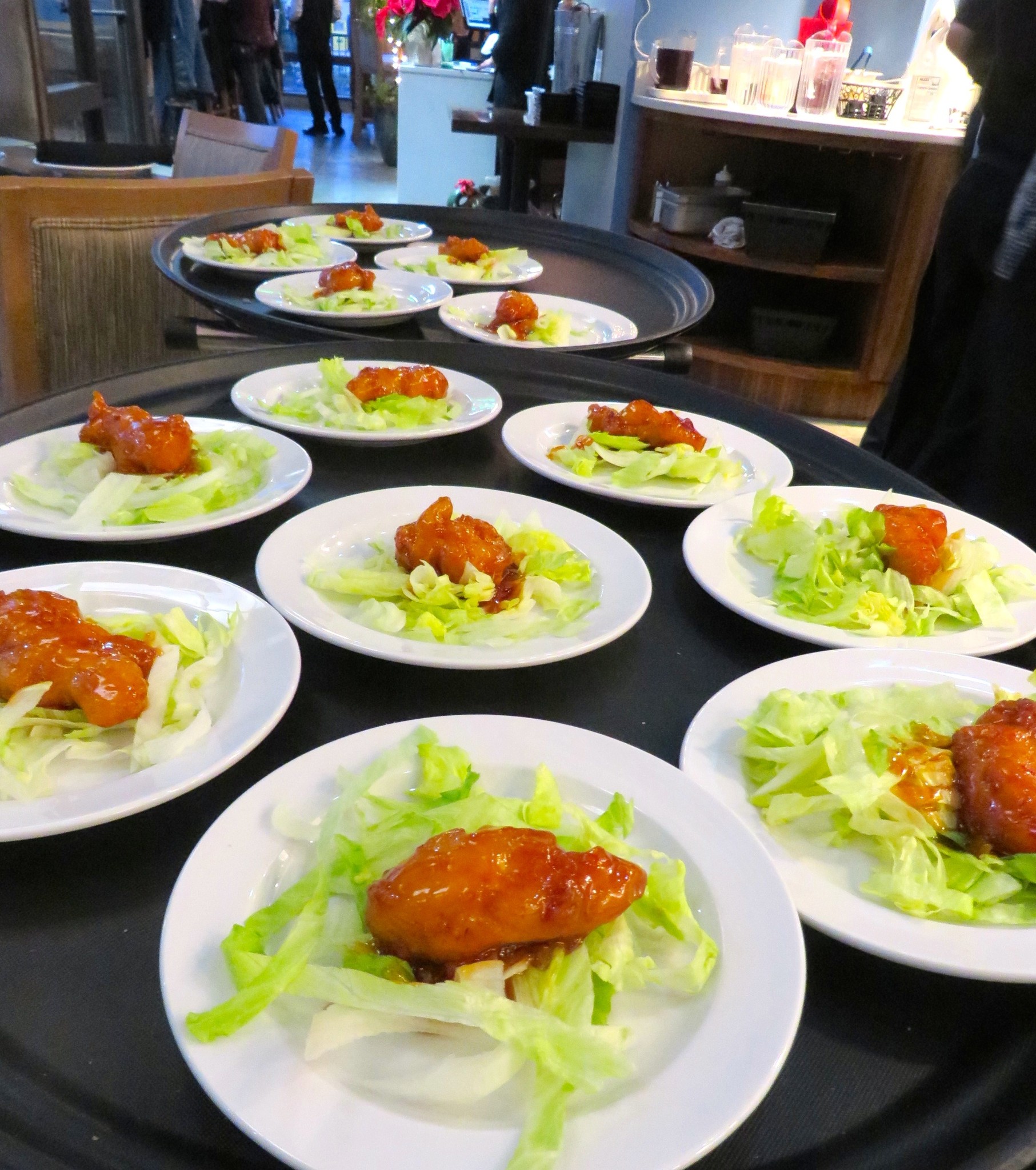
[168,108,298,179]
[0,166,316,413]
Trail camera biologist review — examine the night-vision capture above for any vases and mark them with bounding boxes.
[403,22,443,69]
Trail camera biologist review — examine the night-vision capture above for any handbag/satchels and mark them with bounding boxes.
[270,40,285,69]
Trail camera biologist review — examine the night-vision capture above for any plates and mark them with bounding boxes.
[683,486,1036,656]
[0,416,313,541]
[282,214,433,253]
[374,246,543,292]
[679,648,1036,985]
[255,486,652,670]
[182,235,358,279]
[158,714,806,1170]
[254,269,453,327]
[438,291,638,348]
[501,401,793,508]
[231,360,503,447]
[0,561,302,843]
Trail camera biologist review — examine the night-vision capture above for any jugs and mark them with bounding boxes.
[648,31,696,91]
[709,41,732,95]
[727,23,804,121]
[796,30,853,122]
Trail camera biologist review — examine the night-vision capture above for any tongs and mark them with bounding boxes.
[849,46,873,77]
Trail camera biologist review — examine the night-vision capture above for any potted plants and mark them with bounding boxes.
[355,0,404,168]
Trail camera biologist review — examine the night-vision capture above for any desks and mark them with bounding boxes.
[451,109,616,219]
[396,65,500,209]
[625,59,966,422]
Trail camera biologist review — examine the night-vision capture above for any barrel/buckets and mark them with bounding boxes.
[553,3,600,94]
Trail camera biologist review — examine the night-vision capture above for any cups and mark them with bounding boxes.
[440,39,454,68]
[486,176,501,195]
[476,100,494,123]
[525,91,535,118]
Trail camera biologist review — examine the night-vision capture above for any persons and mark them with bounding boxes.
[291,0,346,139]
[198,0,285,125]
[860,0,1036,538]
[488,0,557,213]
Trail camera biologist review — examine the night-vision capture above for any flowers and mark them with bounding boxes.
[375,0,470,53]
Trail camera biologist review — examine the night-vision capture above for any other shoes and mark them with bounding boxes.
[329,119,345,137]
[303,120,328,135]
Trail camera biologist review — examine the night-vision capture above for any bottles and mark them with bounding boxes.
[715,164,732,188]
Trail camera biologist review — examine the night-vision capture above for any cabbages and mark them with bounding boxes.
[741,675,1035,926]
[14,351,463,522]
[1,601,243,797]
[181,724,720,1170]
[742,494,1017,639]
[176,211,569,342]
[301,430,743,642]
